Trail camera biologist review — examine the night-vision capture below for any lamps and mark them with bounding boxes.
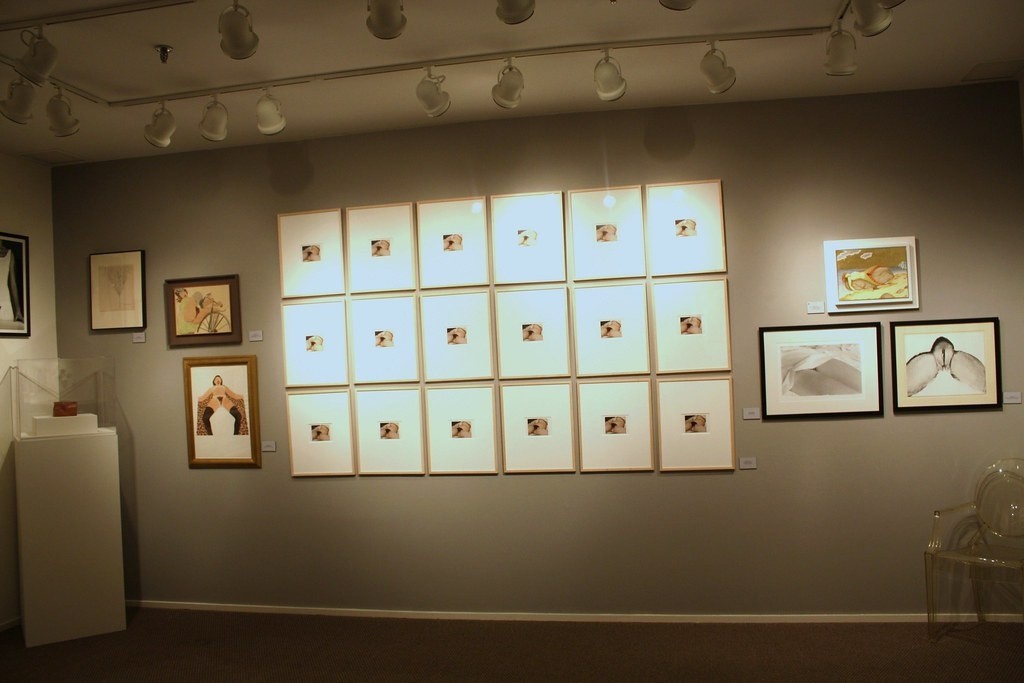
[142,104,179,151]
[0,76,37,126]
[366,0,408,39]
[254,85,287,134]
[494,2,538,25]
[15,29,61,82]
[825,19,858,74]
[492,53,528,112]
[47,89,84,138]
[594,46,627,102]
[416,65,454,119]
[218,0,261,60]
[698,41,739,95]
[197,94,230,141]
[849,1,903,36]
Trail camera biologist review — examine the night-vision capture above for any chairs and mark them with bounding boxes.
[923,458,1024,649]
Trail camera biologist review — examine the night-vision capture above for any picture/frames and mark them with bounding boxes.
[426,388,502,478]
[493,286,572,379]
[651,279,734,378]
[420,292,494,385]
[567,183,646,281]
[581,383,656,472]
[417,197,488,289]
[487,190,564,284]
[348,297,419,384]
[658,380,734,473]
[0,233,264,473]
[351,393,425,480]
[503,381,574,476]
[348,202,415,296]
[281,299,346,388]
[278,394,353,478]
[644,181,729,275]
[276,209,343,298]
[756,236,1006,419]
[572,282,648,379]
